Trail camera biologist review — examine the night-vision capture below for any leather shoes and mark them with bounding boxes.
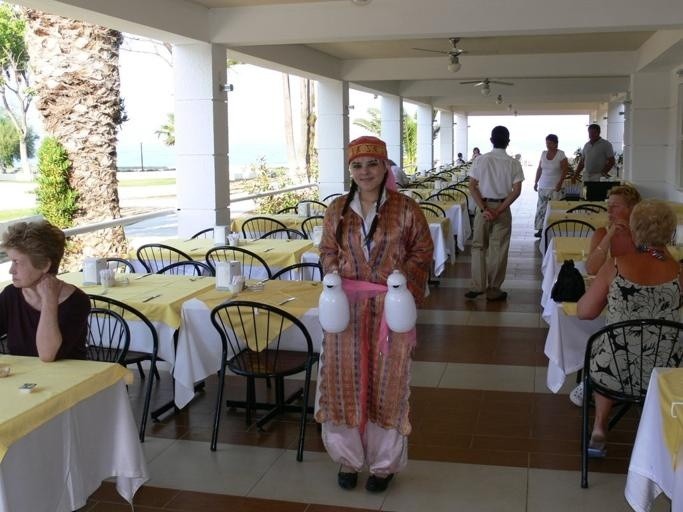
[464,291,484,298]
[366,473,394,492]
[338,463,358,489]
[535,232,542,237]
[486,291,508,301]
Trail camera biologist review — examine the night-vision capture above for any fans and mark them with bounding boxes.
[412,37,468,56]
[458,78,514,86]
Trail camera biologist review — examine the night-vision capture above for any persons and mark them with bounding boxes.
[570,186,640,407]
[471,148,481,160]
[313,137,434,491]
[0,221,91,362]
[576,199,683,457]
[534,135,567,237]
[464,126,525,301]
[571,124,615,184]
[388,160,408,188]
[456,153,465,164]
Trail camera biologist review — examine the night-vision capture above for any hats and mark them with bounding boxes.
[347,136,396,166]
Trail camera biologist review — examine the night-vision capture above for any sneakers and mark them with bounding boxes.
[585,429,610,460]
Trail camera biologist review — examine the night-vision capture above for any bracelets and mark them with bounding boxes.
[481,205,486,213]
[597,246,608,256]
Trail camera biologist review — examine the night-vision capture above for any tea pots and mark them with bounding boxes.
[318,269,350,334]
[383,269,417,333]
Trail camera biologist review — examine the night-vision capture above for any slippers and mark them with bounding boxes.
[569,381,584,407]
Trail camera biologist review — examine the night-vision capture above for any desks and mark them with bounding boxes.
[540,264,681,394]
[129,239,313,281]
[623,367,682,511]
[541,199,681,253]
[540,237,680,308]
[228,213,454,285]
[58,271,213,435]
[301,244,322,282]
[171,278,325,425]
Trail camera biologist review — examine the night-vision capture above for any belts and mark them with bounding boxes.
[482,198,504,202]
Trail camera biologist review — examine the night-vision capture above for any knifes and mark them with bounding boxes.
[141,293,162,302]
[277,297,295,306]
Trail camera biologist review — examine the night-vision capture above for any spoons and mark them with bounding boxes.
[188,276,206,282]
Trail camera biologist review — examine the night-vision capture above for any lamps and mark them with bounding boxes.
[449,55,462,73]
[495,94,505,104]
[481,85,492,96]
[507,104,512,112]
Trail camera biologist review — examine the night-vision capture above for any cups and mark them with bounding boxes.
[228,237,239,247]
[100,269,115,288]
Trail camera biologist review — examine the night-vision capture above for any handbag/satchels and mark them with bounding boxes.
[551,259,585,302]
[552,188,566,201]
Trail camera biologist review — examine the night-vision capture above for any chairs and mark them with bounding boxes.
[578,318,682,490]
[106,257,135,275]
[85,293,167,444]
[80,308,130,366]
[269,261,323,281]
[188,163,479,251]
[211,300,321,461]
[137,243,200,276]
[540,174,610,255]
[156,259,216,278]
[205,246,273,279]
